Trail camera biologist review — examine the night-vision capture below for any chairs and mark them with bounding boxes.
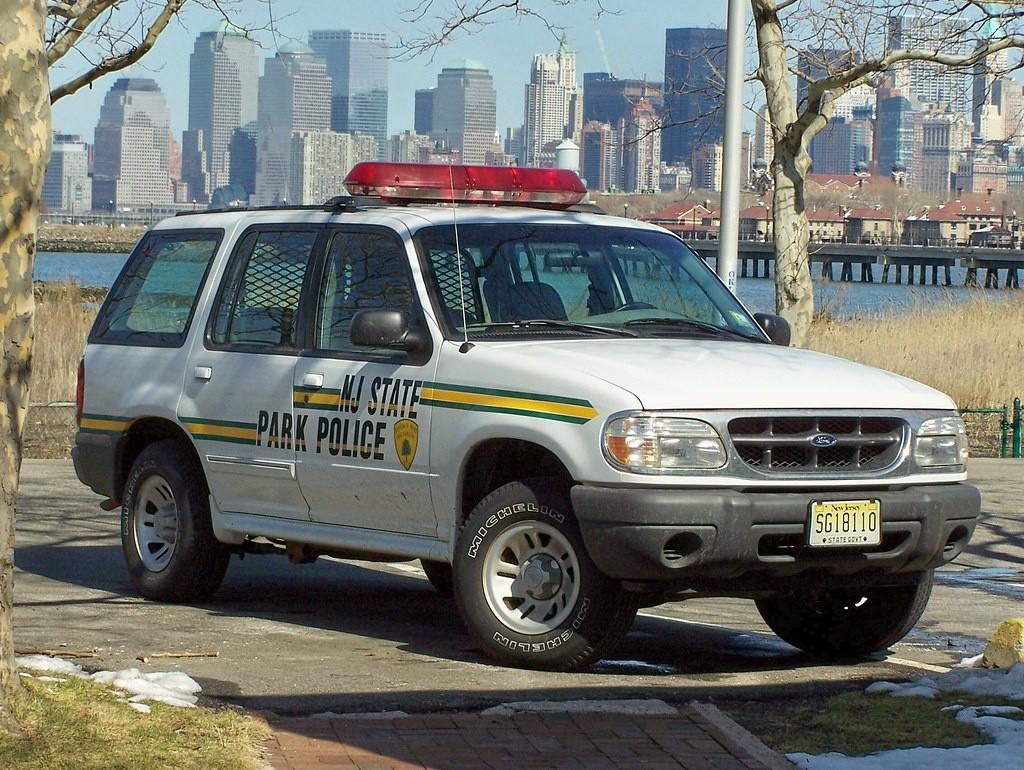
[499,282,568,323]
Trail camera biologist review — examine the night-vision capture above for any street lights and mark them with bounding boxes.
[109,199,114,215]
[842,206,847,245]
[693,205,697,240]
[1010,210,1016,250]
[623,203,629,219]
[150,201,154,226]
[192,197,197,213]
[765,206,770,243]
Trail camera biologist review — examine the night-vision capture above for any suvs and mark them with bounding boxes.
[71,161,982,674]
